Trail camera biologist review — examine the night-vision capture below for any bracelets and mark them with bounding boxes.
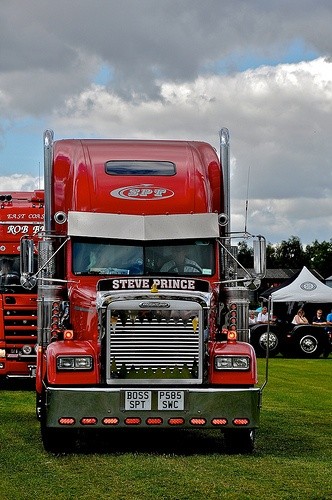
[302,318,304,320]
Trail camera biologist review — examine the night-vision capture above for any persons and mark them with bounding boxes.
[326,313,332,325]
[311,309,327,324]
[257,307,277,324]
[291,308,309,326]
[249,311,258,340]
[160,246,203,272]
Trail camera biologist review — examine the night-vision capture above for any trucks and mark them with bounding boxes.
[0,128,272,453]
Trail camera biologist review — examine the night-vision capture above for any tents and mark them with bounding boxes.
[270,265,332,320]
